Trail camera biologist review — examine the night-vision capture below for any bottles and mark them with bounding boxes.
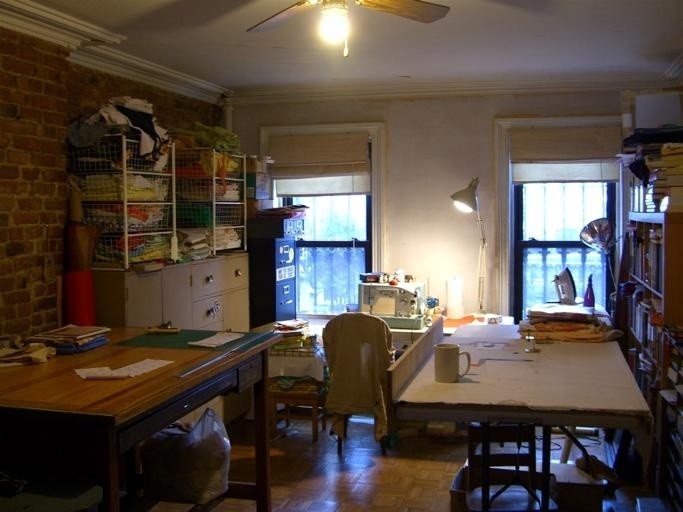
[525,335,536,352]
[583,274,595,307]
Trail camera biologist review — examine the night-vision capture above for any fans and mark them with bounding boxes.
[245,1,451,36]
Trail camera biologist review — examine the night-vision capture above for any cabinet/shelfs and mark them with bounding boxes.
[619,210,683,499]
[649,330,683,512]
[71,130,182,273]
[171,145,251,255]
[87,253,251,438]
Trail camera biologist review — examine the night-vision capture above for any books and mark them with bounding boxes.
[627,223,662,292]
[644,127,682,214]
[634,304,661,360]
[272,318,308,350]
[30,324,112,353]
[660,329,683,510]
[526,303,595,322]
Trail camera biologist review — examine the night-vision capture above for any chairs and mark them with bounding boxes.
[449,420,559,511]
[323,313,403,457]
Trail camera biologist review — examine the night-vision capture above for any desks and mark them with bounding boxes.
[436,311,518,344]
[396,326,651,468]
[1,325,283,512]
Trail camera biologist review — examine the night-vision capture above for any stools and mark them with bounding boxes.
[270,385,327,442]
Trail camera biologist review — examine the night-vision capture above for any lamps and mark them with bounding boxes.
[449,175,487,312]
[318,1,355,57]
[580,218,614,294]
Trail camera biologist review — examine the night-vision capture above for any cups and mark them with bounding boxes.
[346,304,358,312]
[434,343,471,383]
